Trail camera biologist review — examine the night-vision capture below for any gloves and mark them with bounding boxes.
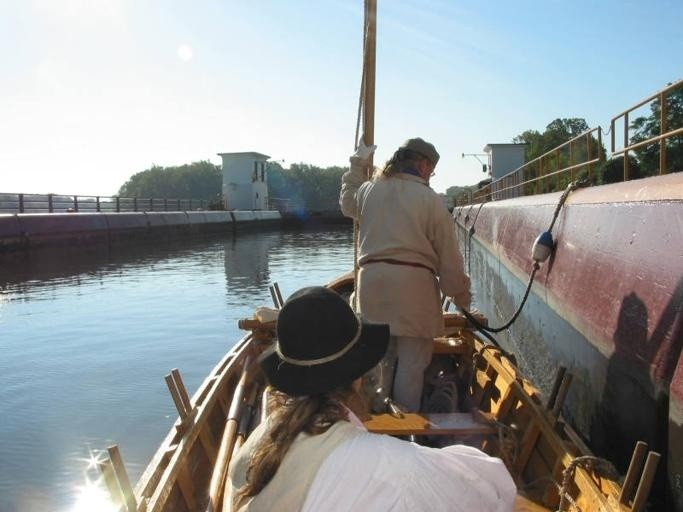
[455,304,470,317]
[355,134,377,161]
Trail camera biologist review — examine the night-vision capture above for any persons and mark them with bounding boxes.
[338,133,472,416]
[224,286,391,512]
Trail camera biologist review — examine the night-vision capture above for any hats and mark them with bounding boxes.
[401,138,440,168]
[258,286,390,397]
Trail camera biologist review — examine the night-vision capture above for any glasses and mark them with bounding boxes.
[430,169,435,177]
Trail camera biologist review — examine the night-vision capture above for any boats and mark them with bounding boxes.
[97,267,664,512]
